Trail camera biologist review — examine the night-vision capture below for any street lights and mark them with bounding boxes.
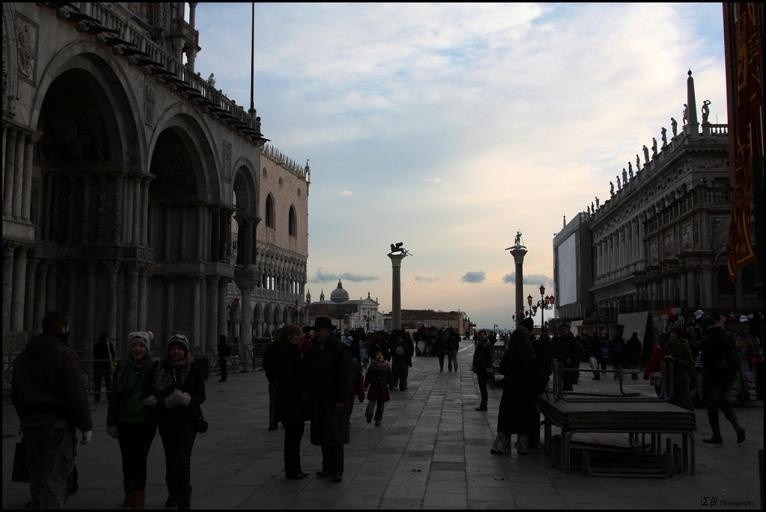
[527,284,555,335]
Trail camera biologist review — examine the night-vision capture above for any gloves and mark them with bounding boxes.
[108,427,118,438]
[141,389,192,413]
[81,431,92,445]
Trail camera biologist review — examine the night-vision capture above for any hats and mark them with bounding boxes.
[168,334,188,349]
[129,331,153,351]
[311,317,337,330]
[518,317,534,331]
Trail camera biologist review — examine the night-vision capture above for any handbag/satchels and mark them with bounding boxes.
[11,442,29,483]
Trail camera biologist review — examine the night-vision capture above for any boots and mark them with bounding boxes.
[121,473,194,510]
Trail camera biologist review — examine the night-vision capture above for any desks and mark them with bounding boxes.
[539,384,698,473]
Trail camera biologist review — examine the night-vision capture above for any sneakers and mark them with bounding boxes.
[439,366,458,373]
[515,443,529,453]
[475,407,487,411]
[217,379,226,382]
[400,387,408,391]
[737,428,745,443]
[491,441,511,454]
[92,399,100,403]
[389,387,398,391]
[256,414,391,483]
[703,435,722,444]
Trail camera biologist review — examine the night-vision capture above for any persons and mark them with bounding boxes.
[11,310,94,510]
[155,333,206,509]
[106,330,158,509]
[92,331,116,404]
[470,329,491,411]
[490,316,746,456]
[261,317,461,483]
[587,98,711,215]
[218,335,228,382]
